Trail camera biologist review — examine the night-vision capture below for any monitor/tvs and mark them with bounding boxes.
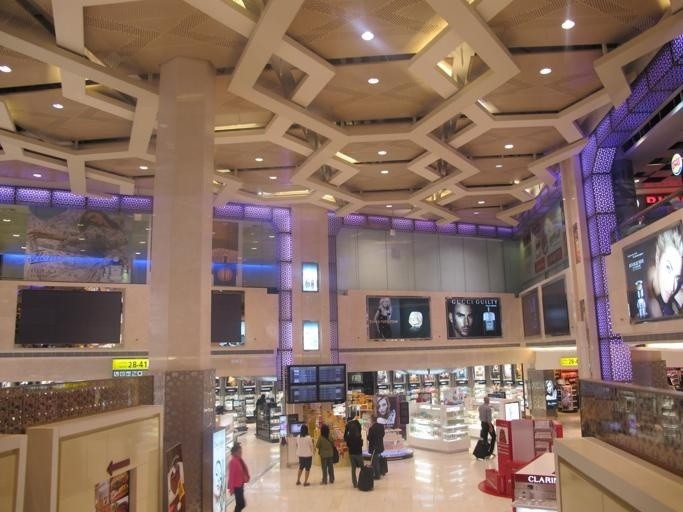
[288,365,318,384]
[317,363,346,384]
[318,384,347,402]
[288,385,318,403]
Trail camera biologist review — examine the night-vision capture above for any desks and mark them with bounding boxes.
[511,452,558,512]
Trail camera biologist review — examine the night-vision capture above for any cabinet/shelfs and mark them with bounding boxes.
[462,397,521,442]
[406,402,471,453]
[217,397,281,458]
[555,376,579,413]
[503,386,525,417]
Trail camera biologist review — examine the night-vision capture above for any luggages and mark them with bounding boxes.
[380,453,388,476]
[358,468,373,491]
[473,440,490,459]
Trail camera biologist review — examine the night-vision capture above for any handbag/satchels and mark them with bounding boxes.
[333,447,339,463]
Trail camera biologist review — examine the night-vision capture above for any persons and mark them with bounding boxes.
[544,379,558,400]
[256,394,266,410]
[478,397,496,456]
[316,424,335,484]
[26,211,127,283]
[295,425,314,486]
[416,392,426,403]
[448,302,473,338]
[375,297,393,338]
[637,228,683,319]
[347,424,364,488]
[344,417,363,466]
[376,397,397,429]
[367,414,385,480]
[228,444,250,512]
